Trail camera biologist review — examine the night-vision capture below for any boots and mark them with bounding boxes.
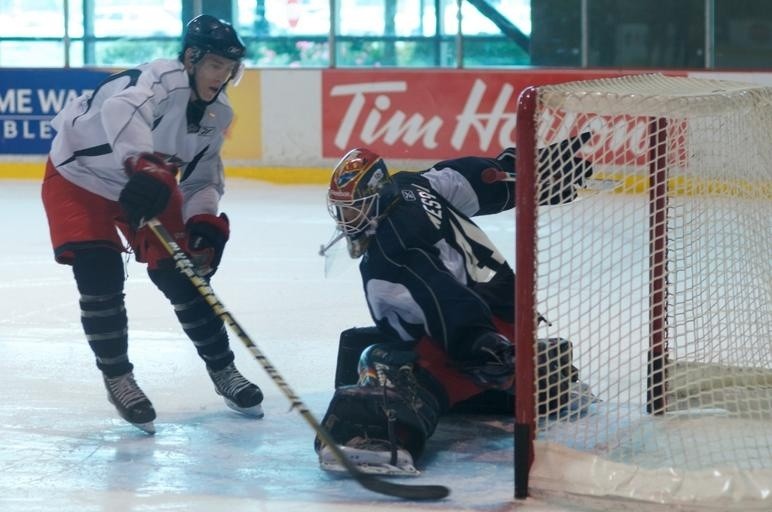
[205,363,265,407]
[101,368,157,424]
[566,379,597,417]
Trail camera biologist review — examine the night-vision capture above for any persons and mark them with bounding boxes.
[313,132,592,468]
[41,14,264,424]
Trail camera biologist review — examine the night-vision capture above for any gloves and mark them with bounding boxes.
[119,162,182,224]
[186,212,230,277]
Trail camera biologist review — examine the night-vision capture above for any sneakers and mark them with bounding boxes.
[321,433,414,464]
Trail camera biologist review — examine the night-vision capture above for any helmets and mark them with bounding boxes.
[327,149,393,206]
[180,14,248,79]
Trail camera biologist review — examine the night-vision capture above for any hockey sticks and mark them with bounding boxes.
[147,215,449,499]
